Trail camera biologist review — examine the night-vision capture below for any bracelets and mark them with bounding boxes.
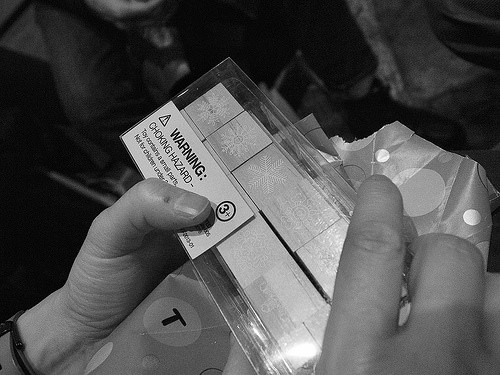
[0,307,39,375]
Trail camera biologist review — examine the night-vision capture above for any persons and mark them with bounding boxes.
[0,173,500,375]
